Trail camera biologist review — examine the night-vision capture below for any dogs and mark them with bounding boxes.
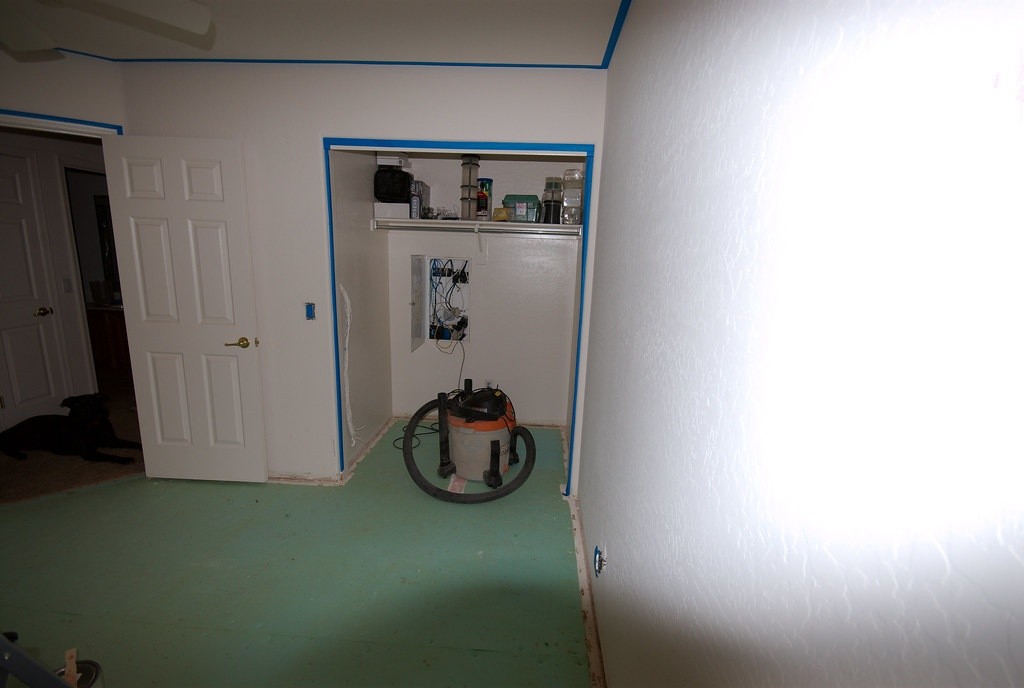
[0,393,142,464]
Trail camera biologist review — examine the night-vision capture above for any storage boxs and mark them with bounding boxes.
[376,151,412,167]
[374,202,410,219]
[410,181,430,219]
[502,195,542,223]
[477,179,493,220]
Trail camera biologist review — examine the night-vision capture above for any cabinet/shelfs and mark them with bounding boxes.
[87,309,133,380]
